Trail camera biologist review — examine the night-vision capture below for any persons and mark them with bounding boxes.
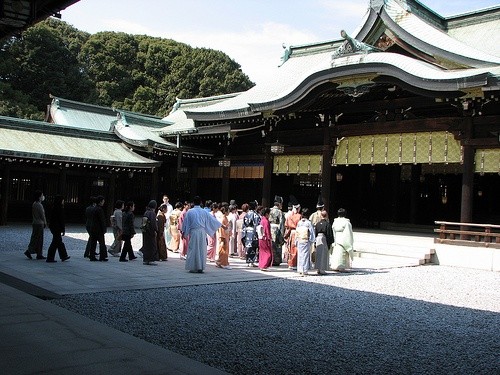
[108,201,125,257]
[119,200,138,261]
[142,200,159,265]
[332,208,353,272]
[156,195,335,274]
[24,191,47,259]
[84,195,109,261]
[137,203,168,261]
[45,196,71,263]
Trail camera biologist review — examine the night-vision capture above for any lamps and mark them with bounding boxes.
[264,139,291,153]
[215,154,231,167]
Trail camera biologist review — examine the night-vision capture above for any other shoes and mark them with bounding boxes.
[89,258,99,261]
[137,251,144,258]
[36,255,47,260]
[24,250,32,259]
[119,258,128,261]
[299,271,308,275]
[46,259,57,262]
[228,253,247,260]
[248,262,259,268]
[99,258,108,261]
[317,270,327,275]
[143,261,157,265]
[260,267,269,271]
[210,258,215,262]
[62,256,70,262]
[108,248,121,257]
[288,265,297,270]
[95,252,99,255]
[156,258,169,261]
[84,254,89,257]
[189,269,205,273]
[180,255,187,260]
[220,264,232,270]
[129,256,138,260]
